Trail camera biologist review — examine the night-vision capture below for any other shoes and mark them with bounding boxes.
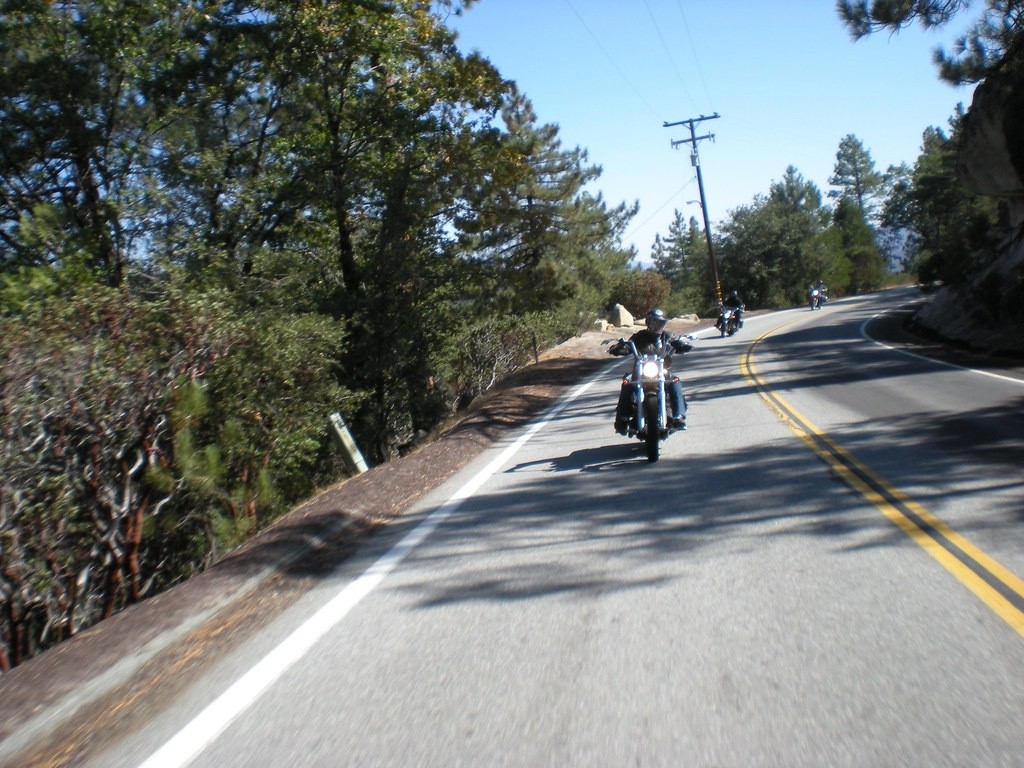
[714,324,720,328]
[673,416,686,429]
[614,420,627,435]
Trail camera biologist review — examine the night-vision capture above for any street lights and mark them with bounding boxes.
[685,200,724,317]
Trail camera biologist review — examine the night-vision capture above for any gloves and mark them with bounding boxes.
[609,344,630,356]
[675,345,692,354]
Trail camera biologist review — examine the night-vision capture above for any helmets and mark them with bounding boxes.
[643,308,667,330]
[816,279,823,285]
[729,290,737,298]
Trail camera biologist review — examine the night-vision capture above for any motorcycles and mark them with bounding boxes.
[809,285,828,311]
[599,332,700,463]
[715,303,747,338]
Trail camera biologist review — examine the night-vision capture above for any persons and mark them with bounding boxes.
[808,279,825,309]
[609,309,692,436]
[714,290,743,332]
